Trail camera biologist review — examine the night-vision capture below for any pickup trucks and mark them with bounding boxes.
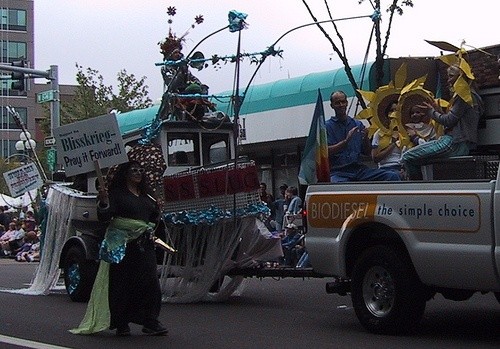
[305,164,500,337]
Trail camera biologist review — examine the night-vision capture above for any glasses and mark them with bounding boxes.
[130,168,145,173]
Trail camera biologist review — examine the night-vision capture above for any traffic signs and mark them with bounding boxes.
[44,135,56,148]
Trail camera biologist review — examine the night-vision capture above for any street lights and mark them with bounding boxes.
[15,131,36,165]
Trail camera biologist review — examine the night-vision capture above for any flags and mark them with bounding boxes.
[298,88,330,185]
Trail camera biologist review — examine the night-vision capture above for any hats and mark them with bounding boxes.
[284,223,298,230]
[280,184,287,189]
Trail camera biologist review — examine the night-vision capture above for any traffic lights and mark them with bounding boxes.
[11,59,27,92]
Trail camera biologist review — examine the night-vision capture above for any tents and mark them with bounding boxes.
[0,187,37,212]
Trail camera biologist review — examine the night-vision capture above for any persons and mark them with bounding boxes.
[259,183,310,268]
[324,91,400,182]
[176,150,188,165]
[157,36,208,94]
[0,203,41,262]
[67,159,169,338]
[401,66,485,181]
[371,88,437,181]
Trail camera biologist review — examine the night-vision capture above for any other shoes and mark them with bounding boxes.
[116,324,131,336]
[142,318,168,336]
